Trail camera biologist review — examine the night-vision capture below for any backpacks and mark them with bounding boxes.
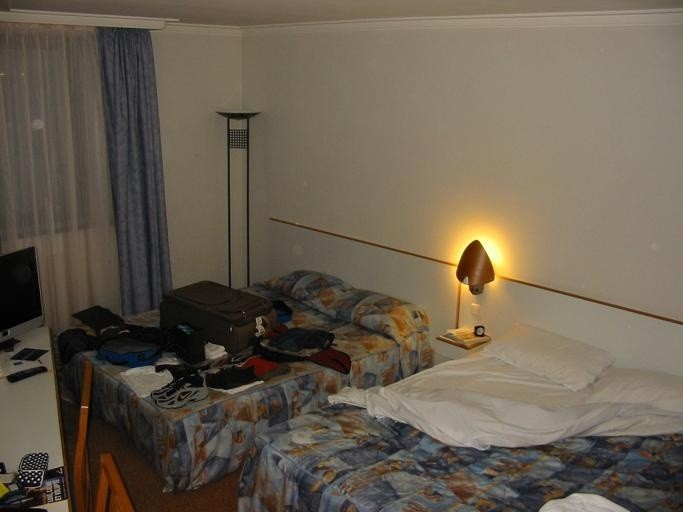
[100,336,159,367]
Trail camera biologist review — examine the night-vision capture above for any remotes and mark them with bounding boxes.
[7,365,49,383]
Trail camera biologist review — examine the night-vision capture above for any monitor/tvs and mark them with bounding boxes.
[0,245,47,353]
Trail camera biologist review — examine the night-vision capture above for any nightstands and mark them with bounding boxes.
[433,333,491,366]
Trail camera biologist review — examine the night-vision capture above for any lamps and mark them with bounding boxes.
[455,241,495,294]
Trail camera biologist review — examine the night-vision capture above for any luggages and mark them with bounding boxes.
[160,281,277,353]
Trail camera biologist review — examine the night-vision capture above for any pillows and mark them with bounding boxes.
[481,323,682,414]
[258,270,430,346]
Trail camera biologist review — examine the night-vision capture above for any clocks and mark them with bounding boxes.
[475,326,484,337]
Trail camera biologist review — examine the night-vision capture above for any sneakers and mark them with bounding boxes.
[151,376,208,409]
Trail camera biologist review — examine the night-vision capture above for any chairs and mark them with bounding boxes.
[66,361,137,512]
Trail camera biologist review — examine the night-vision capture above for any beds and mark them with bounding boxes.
[60,284,432,492]
[250,346,682,512]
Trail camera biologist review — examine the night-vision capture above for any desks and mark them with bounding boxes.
[0,325,72,512]
